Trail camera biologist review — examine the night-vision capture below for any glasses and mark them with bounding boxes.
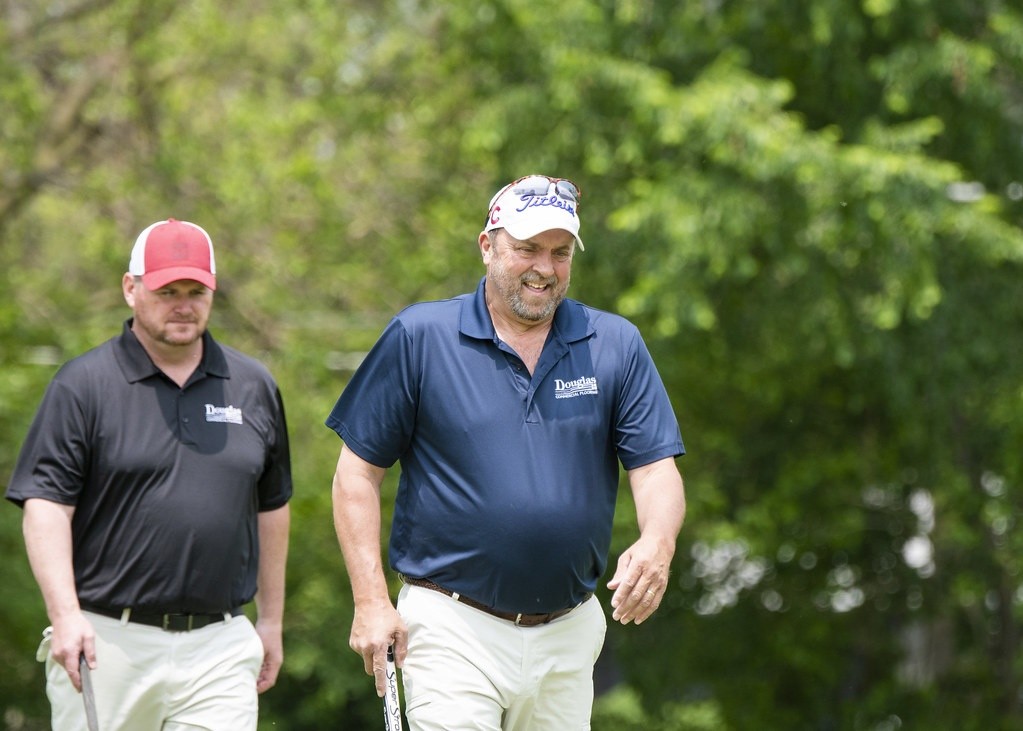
[491,175,583,203]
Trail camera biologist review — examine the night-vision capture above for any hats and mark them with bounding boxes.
[485,177,585,253]
[127,219,216,292]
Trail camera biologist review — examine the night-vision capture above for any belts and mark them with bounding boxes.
[404,574,594,627]
[78,600,246,632]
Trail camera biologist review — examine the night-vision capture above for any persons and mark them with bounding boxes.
[325,174,687,731]
[5,220,293,731]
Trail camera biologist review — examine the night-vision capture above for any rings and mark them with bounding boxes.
[647,590,655,596]
